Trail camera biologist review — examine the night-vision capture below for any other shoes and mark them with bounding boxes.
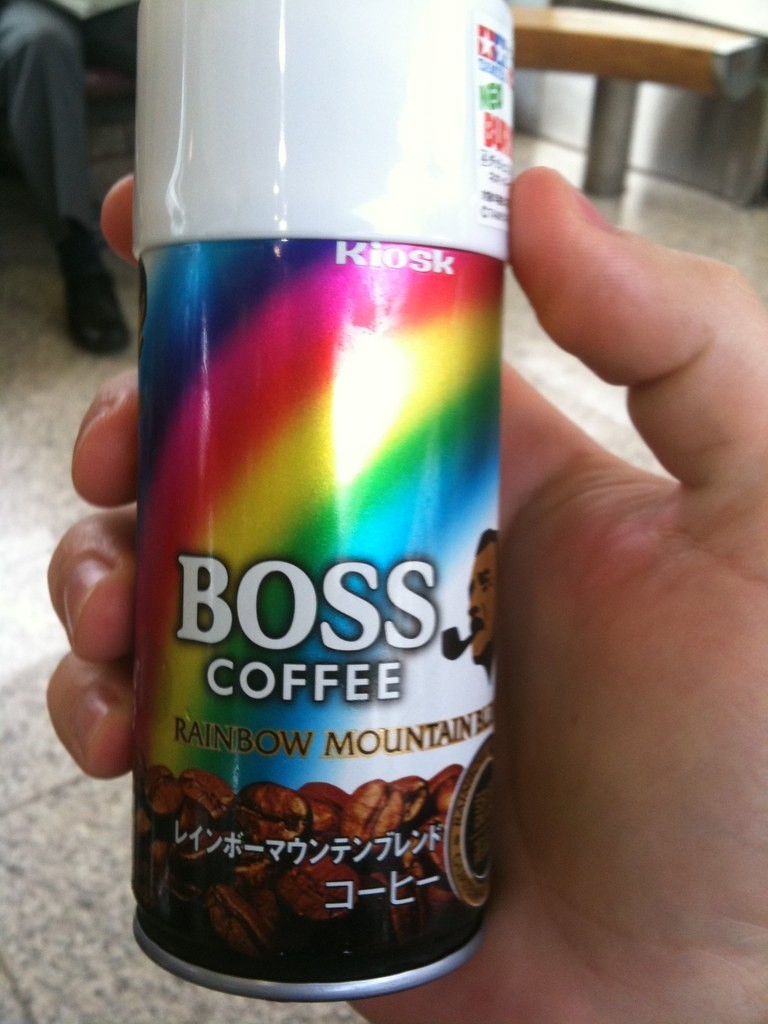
[60,246,129,358]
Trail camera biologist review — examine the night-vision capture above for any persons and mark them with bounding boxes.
[0,0,139,353]
[49,166,768,1024]
